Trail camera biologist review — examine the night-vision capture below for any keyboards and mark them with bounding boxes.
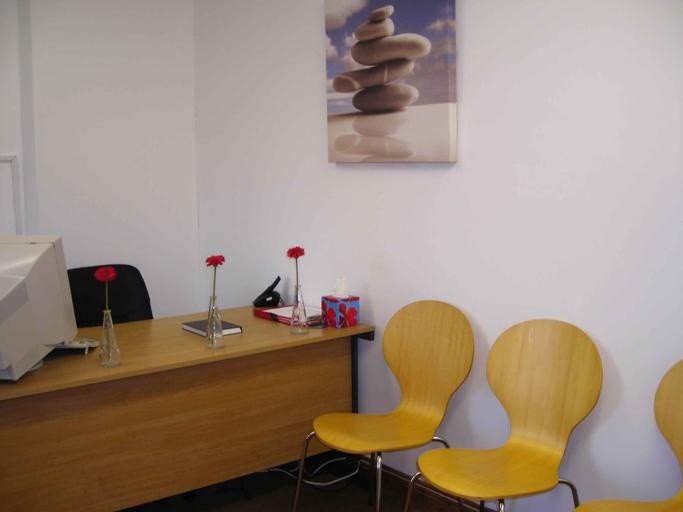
[57,338,100,355]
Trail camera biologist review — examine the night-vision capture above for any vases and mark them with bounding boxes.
[289,284,310,334]
[99,309,121,368]
[203,295,226,348]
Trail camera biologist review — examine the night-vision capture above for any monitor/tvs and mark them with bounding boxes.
[0,234,78,382]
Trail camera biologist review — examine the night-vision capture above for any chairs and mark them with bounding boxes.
[67,264,153,328]
[292,301,474,512]
[404,319,603,512]
[574,360,683,512]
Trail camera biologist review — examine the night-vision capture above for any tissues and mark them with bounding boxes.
[321,276,360,329]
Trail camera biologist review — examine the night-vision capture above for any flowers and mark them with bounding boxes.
[94,267,117,363]
[206,255,225,344]
[287,246,304,332]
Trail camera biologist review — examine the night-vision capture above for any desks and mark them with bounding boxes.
[0,306,375,512]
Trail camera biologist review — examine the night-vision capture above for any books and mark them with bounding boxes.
[182,317,242,338]
[254,306,321,326]
